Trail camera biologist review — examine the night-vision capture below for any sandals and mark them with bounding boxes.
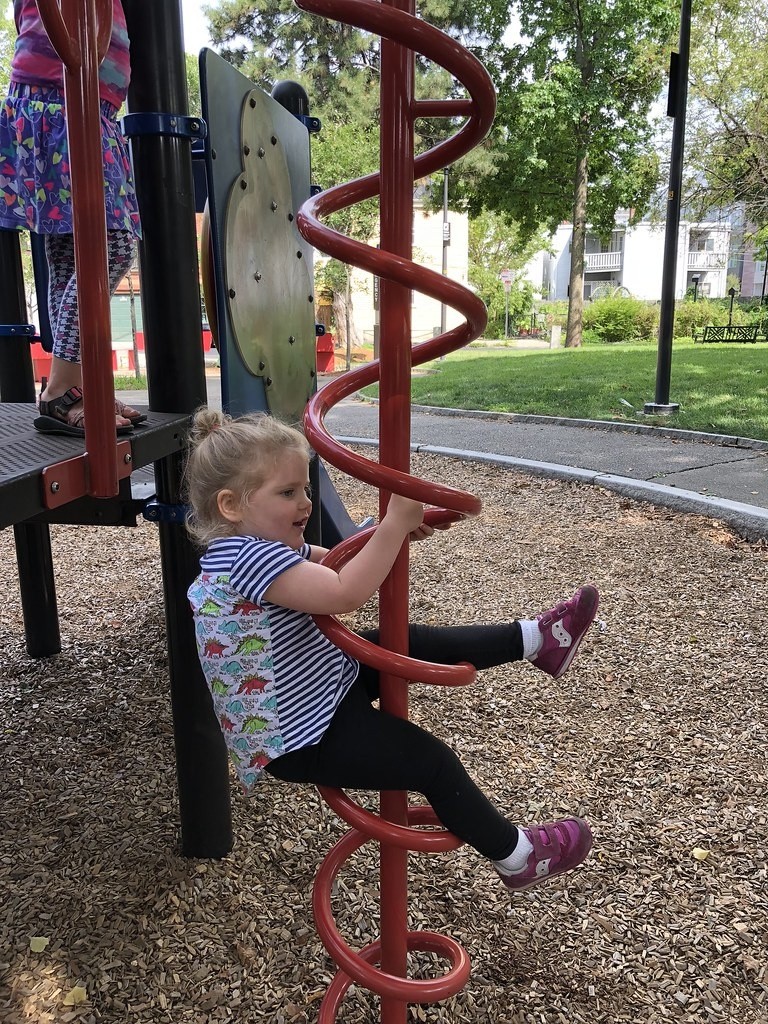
[34,385,147,437]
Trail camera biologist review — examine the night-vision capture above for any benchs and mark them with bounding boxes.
[701,326,758,345]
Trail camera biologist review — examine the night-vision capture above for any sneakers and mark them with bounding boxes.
[491,818,592,890]
[527,585,600,678]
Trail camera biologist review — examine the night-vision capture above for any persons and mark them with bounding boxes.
[184,408,599,890]
[0,0,147,436]
